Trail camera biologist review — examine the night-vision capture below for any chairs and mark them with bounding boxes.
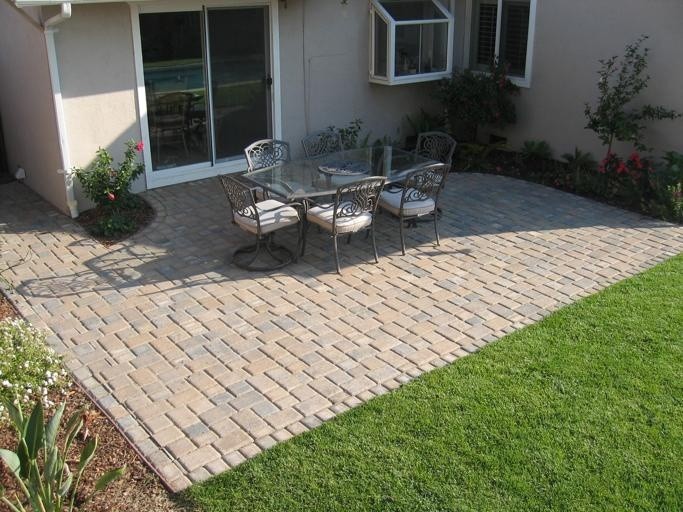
[146,77,204,164]
[216,128,459,277]
